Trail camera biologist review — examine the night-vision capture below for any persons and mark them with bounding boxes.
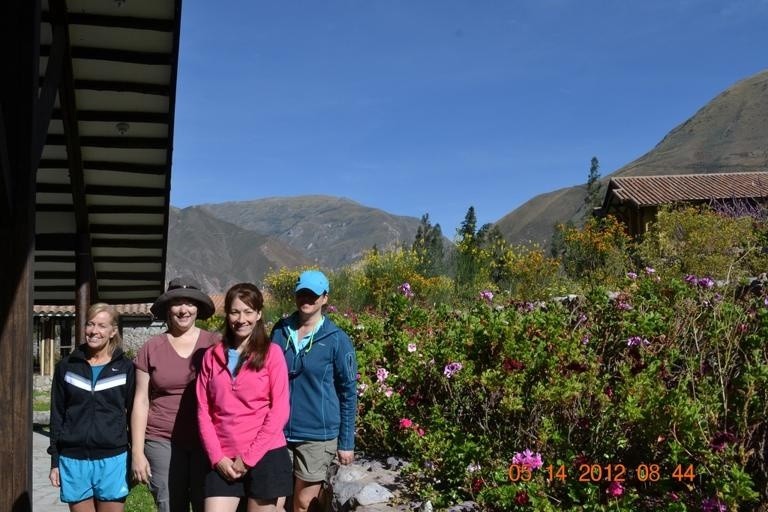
[47,302,139,512]
[130,277,224,512]
[269,270,357,512]
[195,284,294,512]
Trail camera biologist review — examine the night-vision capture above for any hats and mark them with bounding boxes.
[294,271,330,296]
[151,278,215,320]
